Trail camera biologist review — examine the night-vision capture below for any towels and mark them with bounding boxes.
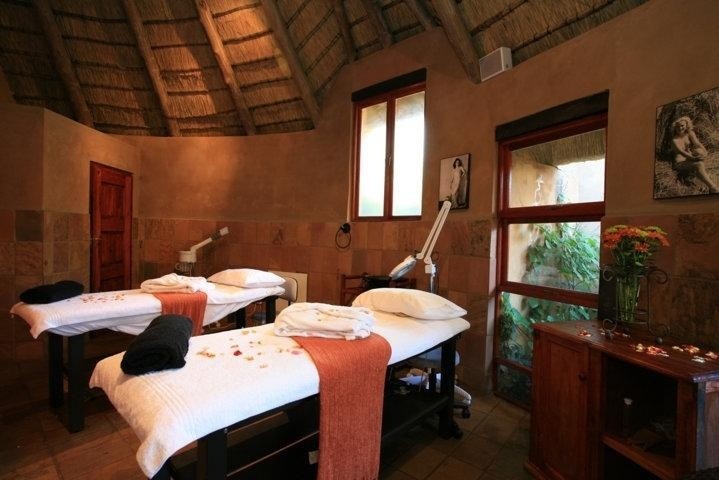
[17,279,85,304]
[121,314,193,376]
[293,334,392,480]
[154,292,207,335]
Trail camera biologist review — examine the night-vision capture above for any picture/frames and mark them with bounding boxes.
[653,84,719,201]
[438,154,470,211]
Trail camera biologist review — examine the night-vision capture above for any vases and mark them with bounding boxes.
[601,264,670,336]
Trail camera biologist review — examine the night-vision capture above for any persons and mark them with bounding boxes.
[448,158,466,205]
[670,116,719,194]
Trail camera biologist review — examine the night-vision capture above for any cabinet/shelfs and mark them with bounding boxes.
[340,272,417,308]
[523,317,719,480]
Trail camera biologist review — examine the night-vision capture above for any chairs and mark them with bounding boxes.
[251,274,298,324]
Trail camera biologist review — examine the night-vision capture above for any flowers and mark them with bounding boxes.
[601,224,671,321]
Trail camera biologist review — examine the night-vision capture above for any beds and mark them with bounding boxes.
[85,290,473,480]
[6,267,286,433]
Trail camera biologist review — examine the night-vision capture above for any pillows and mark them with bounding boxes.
[351,288,468,320]
[207,269,286,288]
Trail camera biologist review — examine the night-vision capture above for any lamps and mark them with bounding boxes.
[389,200,452,292]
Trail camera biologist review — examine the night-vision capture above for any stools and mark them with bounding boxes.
[400,346,472,419]
